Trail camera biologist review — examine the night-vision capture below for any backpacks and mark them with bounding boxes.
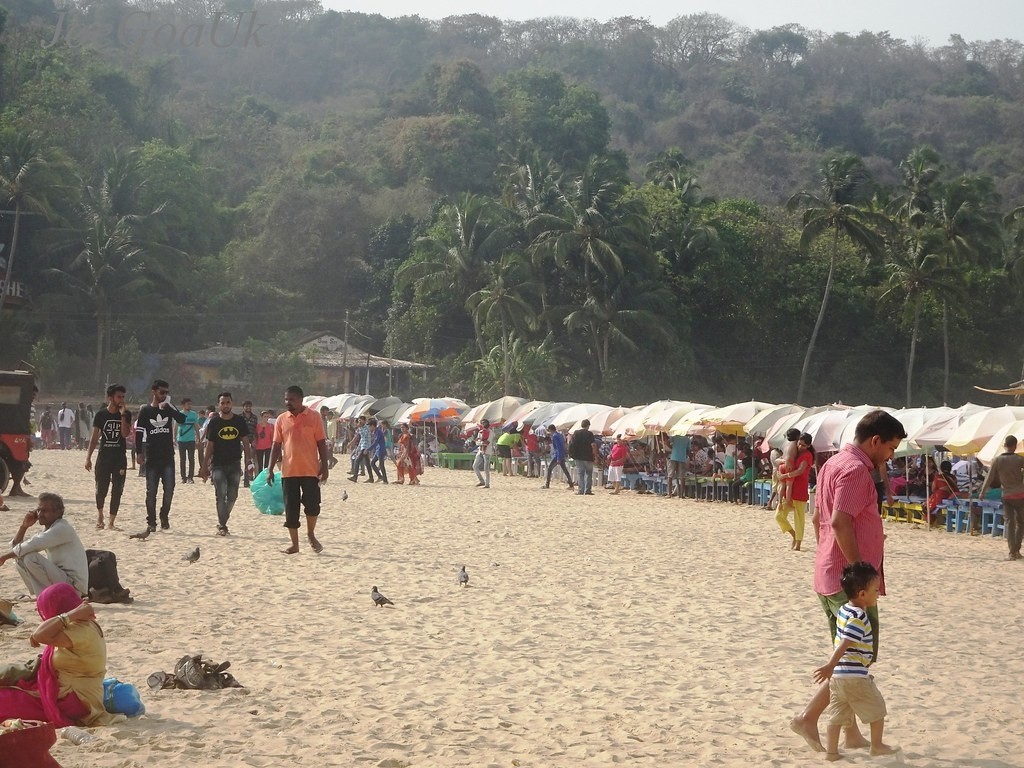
[41,412,51,430]
[85,549,134,603]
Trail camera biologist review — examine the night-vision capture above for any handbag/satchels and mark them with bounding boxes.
[103,678,145,716]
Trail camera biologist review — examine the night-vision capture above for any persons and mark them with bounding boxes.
[9,380,276,537]
[318,406,1024,559]
[0,493,90,606]
[0,582,108,729]
[788,409,909,763]
[266,387,325,556]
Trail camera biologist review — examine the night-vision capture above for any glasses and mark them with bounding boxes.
[156,390,168,395]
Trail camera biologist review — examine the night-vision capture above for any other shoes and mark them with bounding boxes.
[147,519,157,531]
[159,514,170,529]
[0,505,9,511]
[567,486,573,490]
[541,485,549,489]
[1009,550,1022,561]
[477,482,484,486]
[585,492,595,495]
[609,491,619,495]
[347,469,419,485]
[216,525,230,536]
[147,653,245,691]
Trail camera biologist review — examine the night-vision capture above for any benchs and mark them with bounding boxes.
[513,456,818,515]
[882,495,1010,538]
[430,452,500,470]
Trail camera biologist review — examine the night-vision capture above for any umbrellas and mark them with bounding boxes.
[296,393,1024,536]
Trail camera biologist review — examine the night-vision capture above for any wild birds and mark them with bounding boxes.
[180,546,202,565]
[371,585,395,608]
[341,489,348,502]
[458,565,469,587]
[129,526,152,541]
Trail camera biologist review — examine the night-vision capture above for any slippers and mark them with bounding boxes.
[280,545,300,554]
[4,594,38,604]
[109,525,121,531]
[97,519,105,530]
[308,537,323,553]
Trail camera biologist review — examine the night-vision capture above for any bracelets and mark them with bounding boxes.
[57,612,71,626]
